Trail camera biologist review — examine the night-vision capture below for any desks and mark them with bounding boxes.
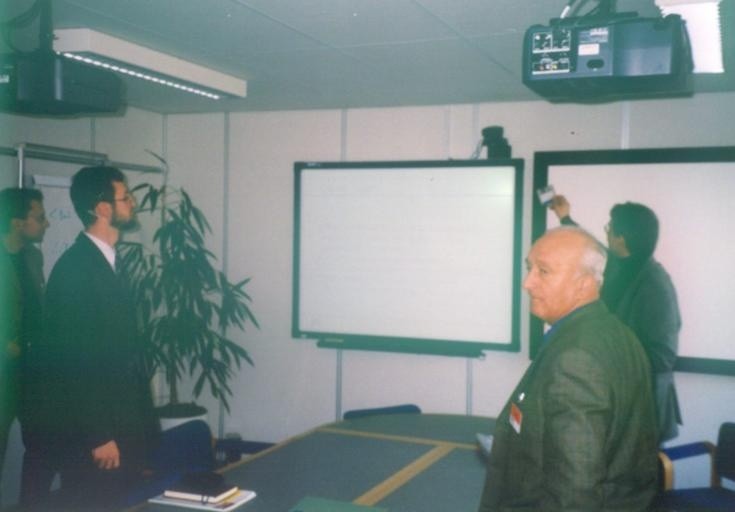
[123,411,499,512]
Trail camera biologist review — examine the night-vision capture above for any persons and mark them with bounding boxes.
[1,187,50,511]
[477,227,659,512]
[37,165,161,511]
[546,193,684,449]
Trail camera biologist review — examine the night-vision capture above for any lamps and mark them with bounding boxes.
[50,24,249,105]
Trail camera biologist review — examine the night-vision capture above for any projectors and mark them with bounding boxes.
[0,47,127,119]
[521,15,697,103]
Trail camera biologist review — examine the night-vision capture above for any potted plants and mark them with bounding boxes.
[114,143,260,431]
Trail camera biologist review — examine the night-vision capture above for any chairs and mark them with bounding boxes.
[160,417,232,477]
[663,418,735,512]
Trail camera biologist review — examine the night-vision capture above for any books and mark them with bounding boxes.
[148,478,257,512]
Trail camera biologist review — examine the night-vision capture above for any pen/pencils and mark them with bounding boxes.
[323,337,344,343]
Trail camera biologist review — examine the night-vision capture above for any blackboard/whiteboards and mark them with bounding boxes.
[292,159,524,357]
[531,146,735,375]
